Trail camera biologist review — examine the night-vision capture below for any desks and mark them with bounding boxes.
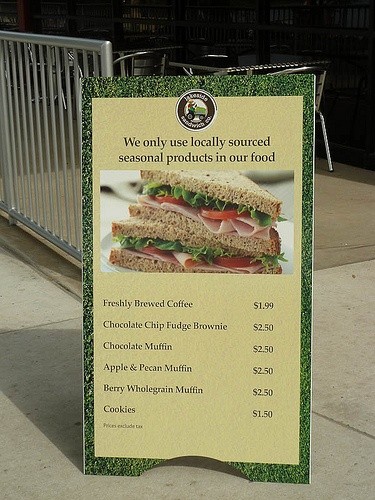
[168,54,333,75]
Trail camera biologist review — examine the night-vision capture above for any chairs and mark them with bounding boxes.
[113,51,169,76]
[1,26,85,110]
[264,64,335,174]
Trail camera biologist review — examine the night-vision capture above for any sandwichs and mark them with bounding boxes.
[106,168,288,274]
